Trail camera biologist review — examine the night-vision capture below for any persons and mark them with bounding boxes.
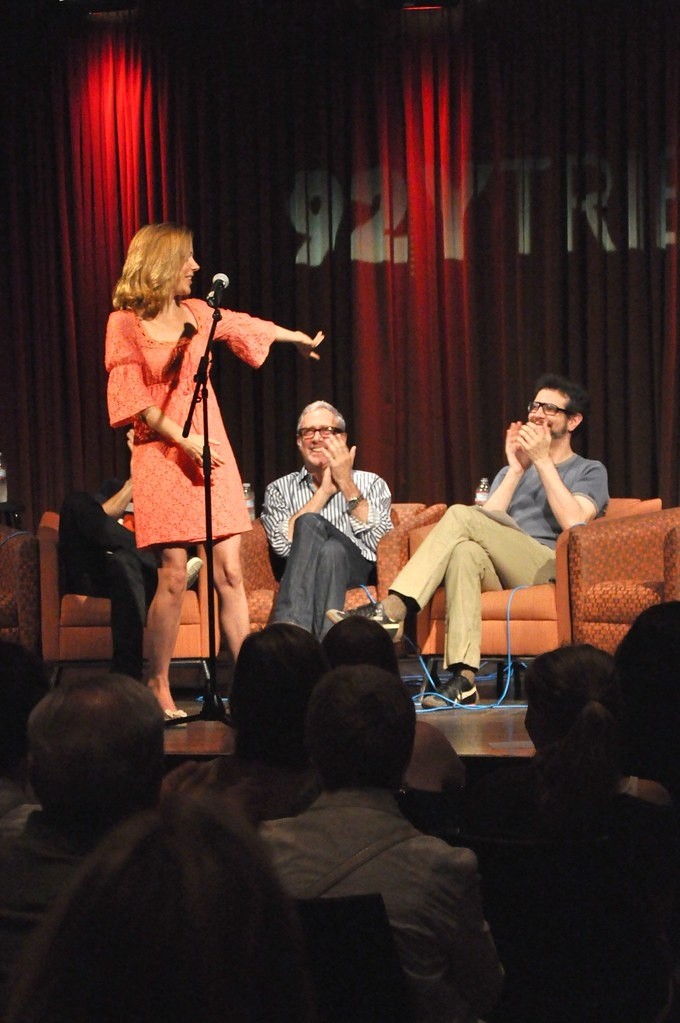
[524,644,672,807]
[321,614,466,794]
[0,672,318,1023]
[612,601,680,795]
[259,663,506,1023]
[259,400,394,643]
[0,639,53,819]
[157,623,332,828]
[58,475,203,682]
[104,222,326,727]
[325,374,610,709]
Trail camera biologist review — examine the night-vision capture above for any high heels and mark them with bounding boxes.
[161,708,188,728]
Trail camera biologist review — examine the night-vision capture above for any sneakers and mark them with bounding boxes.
[326,601,405,643]
[422,673,480,709]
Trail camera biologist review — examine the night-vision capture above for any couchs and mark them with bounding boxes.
[568,507,680,656]
[409,498,663,702]
[239,503,447,695]
[0,525,39,681]
[41,508,221,709]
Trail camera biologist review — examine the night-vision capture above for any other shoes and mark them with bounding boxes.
[185,555,203,588]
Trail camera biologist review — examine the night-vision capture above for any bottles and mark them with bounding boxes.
[241,483,257,522]
[0,452,8,503]
[475,478,490,507]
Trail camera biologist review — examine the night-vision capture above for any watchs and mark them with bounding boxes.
[348,494,364,510]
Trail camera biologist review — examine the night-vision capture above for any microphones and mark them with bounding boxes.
[206,273,230,301]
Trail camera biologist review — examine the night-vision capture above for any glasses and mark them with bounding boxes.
[527,400,577,416]
[298,426,345,440]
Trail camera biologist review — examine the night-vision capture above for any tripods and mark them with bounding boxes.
[163,299,231,728]
